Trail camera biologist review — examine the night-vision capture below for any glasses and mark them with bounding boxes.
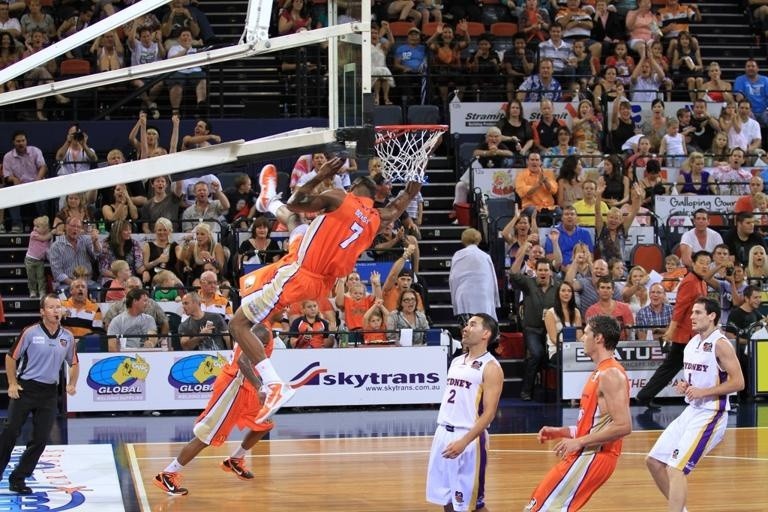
[402,298,416,303]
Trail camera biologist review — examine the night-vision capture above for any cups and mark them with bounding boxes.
[84,223,93,234]
[119,338,126,349]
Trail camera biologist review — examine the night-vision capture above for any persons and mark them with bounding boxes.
[0,1,767,403]
[152,304,288,496]
[1,293,79,493]
[519,312,633,511]
[644,295,746,511]
[228,155,423,424]
[425,313,505,511]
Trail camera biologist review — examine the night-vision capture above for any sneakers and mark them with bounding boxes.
[150,103,160,119]
[451,218,459,225]
[40,293,46,299]
[152,470,189,497]
[255,164,283,213]
[0,222,23,233]
[254,382,296,423]
[520,390,532,400]
[30,292,37,298]
[219,456,255,480]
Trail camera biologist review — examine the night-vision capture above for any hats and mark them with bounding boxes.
[407,27,421,35]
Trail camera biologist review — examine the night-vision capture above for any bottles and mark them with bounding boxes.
[627,325,635,341]
[646,321,654,340]
[715,182,720,195]
[662,150,667,166]
[339,325,347,346]
[98,218,105,234]
[160,248,168,269]
[115,334,120,351]
[671,182,678,196]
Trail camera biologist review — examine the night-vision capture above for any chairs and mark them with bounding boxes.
[371,105,440,127]
[390,23,517,36]
[707,211,729,230]
[459,143,482,175]
[76,330,108,352]
[667,212,695,235]
[485,193,515,240]
[157,312,181,351]
[217,172,291,204]
[542,335,561,403]
[56,57,96,120]
[629,244,666,274]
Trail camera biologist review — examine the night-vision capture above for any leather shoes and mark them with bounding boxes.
[635,392,661,408]
[730,402,739,408]
[9,482,32,493]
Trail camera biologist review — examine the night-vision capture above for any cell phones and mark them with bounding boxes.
[205,319,214,331]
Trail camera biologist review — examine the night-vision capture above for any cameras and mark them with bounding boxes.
[72,132,83,140]
[727,268,734,276]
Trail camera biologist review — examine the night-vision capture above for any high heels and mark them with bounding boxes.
[56,97,69,104]
[36,114,48,122]
